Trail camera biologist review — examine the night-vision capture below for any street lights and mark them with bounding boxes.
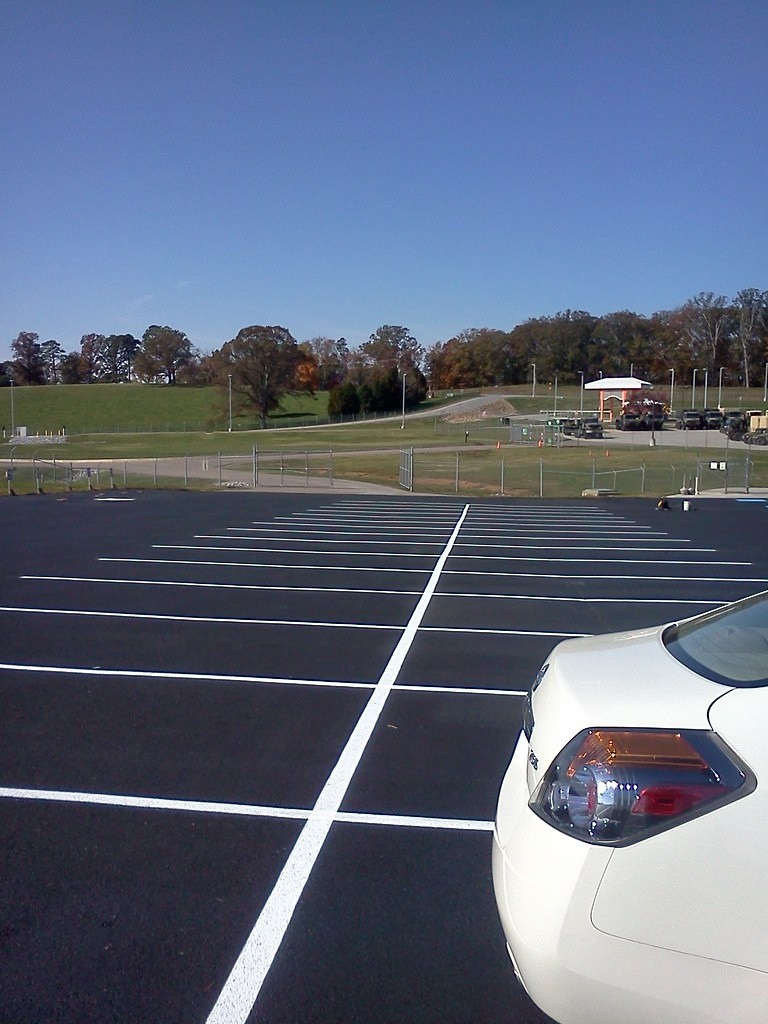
[669,368,674,411]
[718,367,726,409]
[531,363,536,399]
[703,368,708,409]
[9,379,15,437]
[402,374,410,431]
[577,370,584,416]
[553,373,559,415]
[692,369,701,411]
[228,374,234,431]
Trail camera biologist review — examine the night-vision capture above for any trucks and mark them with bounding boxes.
[675,409,768,445]
[561,417,602,439]
[615,405,665,431]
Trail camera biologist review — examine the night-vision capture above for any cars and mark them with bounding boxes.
[492,588,768,1022]
